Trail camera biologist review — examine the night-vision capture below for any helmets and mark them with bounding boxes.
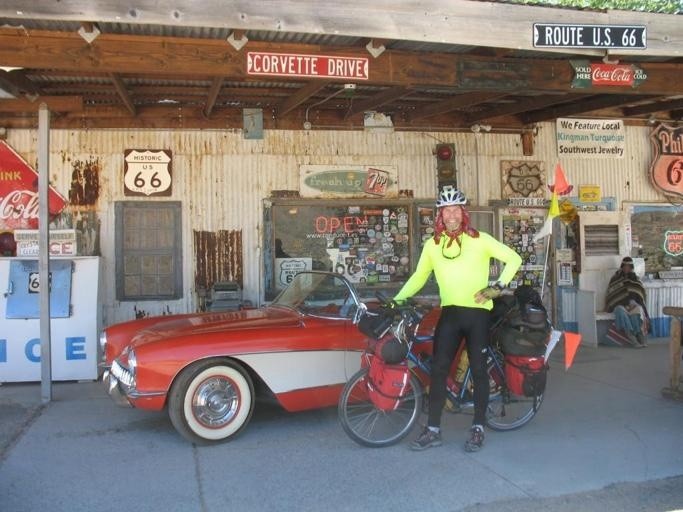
[435,185,467,208]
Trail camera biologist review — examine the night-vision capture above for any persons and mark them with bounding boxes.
[389,185,522,453]
[604,256,648,348]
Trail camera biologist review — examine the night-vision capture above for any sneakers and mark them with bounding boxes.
[407,425,443,451]
[462,425,485,454]
[633,342,647,348]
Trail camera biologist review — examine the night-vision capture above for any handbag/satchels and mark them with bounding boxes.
[504,356,547,398]
[366,335,411,410]
[357,307,394,338]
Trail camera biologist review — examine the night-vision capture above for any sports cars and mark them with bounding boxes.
[96,267,539,451]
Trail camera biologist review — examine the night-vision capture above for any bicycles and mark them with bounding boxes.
[336,290,550,451]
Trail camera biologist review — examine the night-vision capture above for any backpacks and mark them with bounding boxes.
[497,285,548,357]
